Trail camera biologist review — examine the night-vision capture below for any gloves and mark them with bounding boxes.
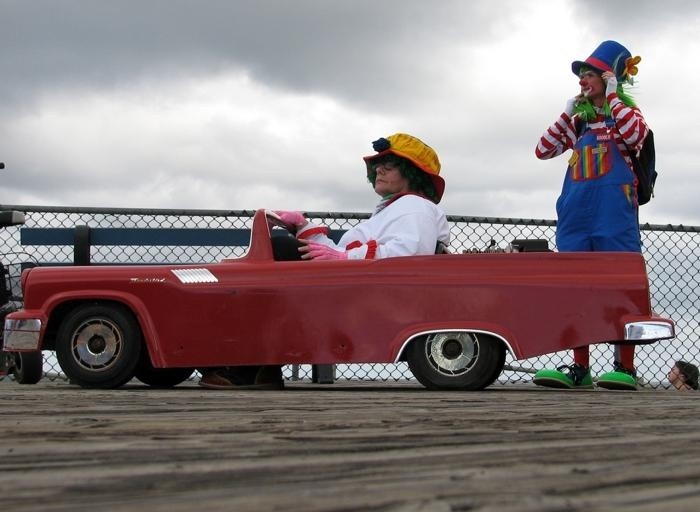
[265,210,309,235]
[298,238,349,262]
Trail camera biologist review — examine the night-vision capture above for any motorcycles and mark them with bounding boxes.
[0,161,44,386]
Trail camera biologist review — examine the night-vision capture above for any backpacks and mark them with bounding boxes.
[623,128,658,205]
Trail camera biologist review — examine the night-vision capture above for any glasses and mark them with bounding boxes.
[372,158,402,171]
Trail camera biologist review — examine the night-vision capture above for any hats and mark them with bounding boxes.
[571,40,641,91]
[363,133,446,204]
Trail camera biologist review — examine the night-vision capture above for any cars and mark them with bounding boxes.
[0,207,675,390]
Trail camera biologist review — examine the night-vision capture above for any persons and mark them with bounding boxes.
[667,361,699,392]
[530,64,651,392]
[196,134,452,391]
